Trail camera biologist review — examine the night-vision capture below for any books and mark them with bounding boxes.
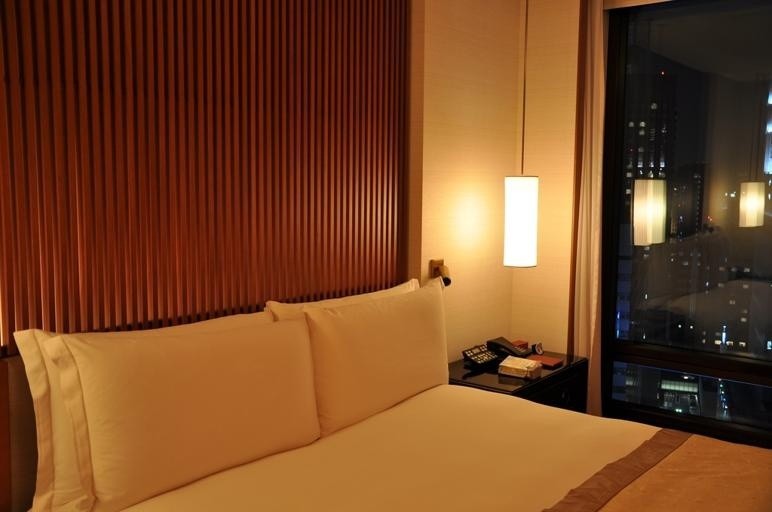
[498,355,542,378]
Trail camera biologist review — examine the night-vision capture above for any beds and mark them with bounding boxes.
[0,279,769,512]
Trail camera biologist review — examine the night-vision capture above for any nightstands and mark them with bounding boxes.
[447,345,588,415]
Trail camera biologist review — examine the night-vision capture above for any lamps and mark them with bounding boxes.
[632,177,667,248]
[429,258,451,287]
[503,0,538,269]
[737,178,765,228]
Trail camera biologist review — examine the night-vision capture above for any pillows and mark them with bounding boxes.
[9,305,277,512]
[49,317,324,512]
[295,276,447,432]
[267,278,422,321]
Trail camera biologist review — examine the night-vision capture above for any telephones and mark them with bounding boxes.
[486,336,532,358]
